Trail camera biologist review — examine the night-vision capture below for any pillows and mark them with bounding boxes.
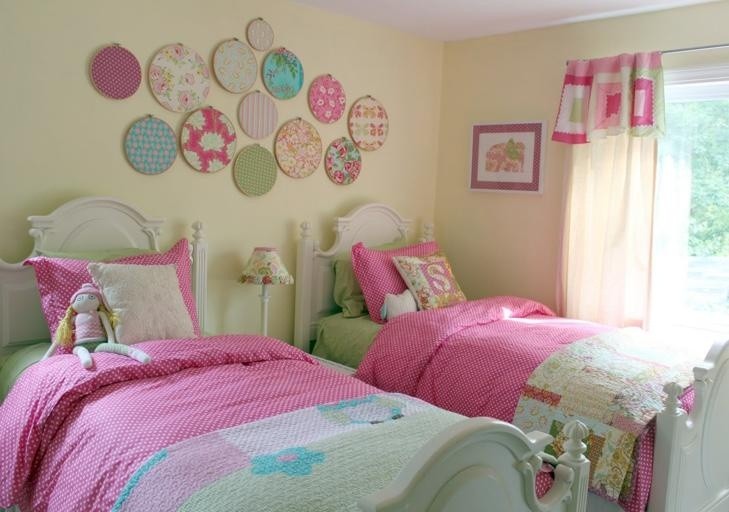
[391,250,469,309]
[326,236,407,320]
[84,256,194,347]
[18,235,207,356]
[31,245,159,260]
[348,237,446,326]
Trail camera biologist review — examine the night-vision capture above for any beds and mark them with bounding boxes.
[293,198,729,512]
[1,196,603,512]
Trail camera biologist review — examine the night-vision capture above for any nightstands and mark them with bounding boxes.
[304,352,358,377]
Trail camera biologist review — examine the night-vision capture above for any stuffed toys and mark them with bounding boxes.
[36,282,151,370]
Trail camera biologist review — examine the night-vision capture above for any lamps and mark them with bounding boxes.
[236,247,300,339]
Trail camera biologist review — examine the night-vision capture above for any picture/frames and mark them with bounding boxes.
[465,119,548,196]
